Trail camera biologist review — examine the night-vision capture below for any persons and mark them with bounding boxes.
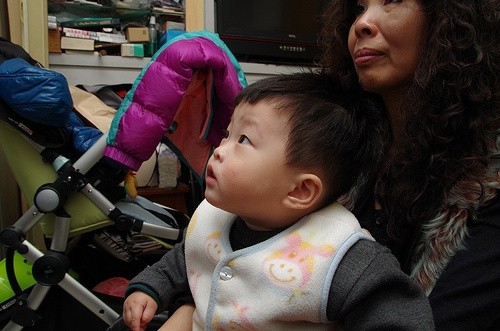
[119,66,435,331]
[153,0,500,331]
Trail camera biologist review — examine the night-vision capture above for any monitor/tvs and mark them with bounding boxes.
[213,0,333,68]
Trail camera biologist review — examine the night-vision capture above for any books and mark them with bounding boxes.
[47,0,185,59]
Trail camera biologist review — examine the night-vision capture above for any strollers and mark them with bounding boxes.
[0,35,200,331]
